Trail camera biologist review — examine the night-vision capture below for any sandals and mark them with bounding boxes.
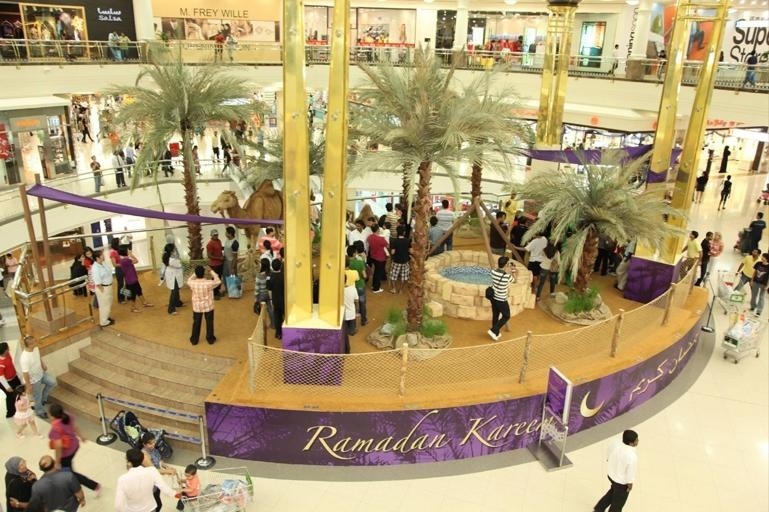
[131,301,155,314]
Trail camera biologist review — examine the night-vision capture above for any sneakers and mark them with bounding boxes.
[100,317,115,327]
[168,302,187,316]
[487,330,503,341]
[592,263,624,292]
[535,292,556,302]
[16,432,44,439]
[345,321,371,337]
[365,283,403,295]
[31,400,52,418]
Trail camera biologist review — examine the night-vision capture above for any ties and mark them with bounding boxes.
[117,157,121,166]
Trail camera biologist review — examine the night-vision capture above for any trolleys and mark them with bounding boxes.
[714,267,748,316]
[720,308,769,364]
[161,463,256,512]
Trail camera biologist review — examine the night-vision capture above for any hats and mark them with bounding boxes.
[210,229,218,238]
[498,257,510,268]
[119,288,132,297]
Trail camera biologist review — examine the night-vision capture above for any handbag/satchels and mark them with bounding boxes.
[225,274,243,299]
[50,422,70,448]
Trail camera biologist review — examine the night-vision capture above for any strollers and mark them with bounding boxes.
[756,190,769,206]
[107,410,175,461]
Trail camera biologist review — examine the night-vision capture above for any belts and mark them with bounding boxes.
[98,284,112,287]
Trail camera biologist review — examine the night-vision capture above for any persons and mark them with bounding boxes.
[593,430,640,511]
[656,49,667,80]
[5,253,19,280]
[488,37,523,65]
[682,147,769,316]
[309,94,328,134]
[52,8,83,56]
[608,44,621,77]
[108,32,132,61]
[68,227,285,344]
[73,101,266,193]
[742,50,758,88]
[154,18,253,40]
[337,198,636,354]
[1,334,202,511]
[213,32,238,62]
[568,134,598,152]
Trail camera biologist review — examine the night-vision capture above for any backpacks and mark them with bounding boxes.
[485,287,496,299]
